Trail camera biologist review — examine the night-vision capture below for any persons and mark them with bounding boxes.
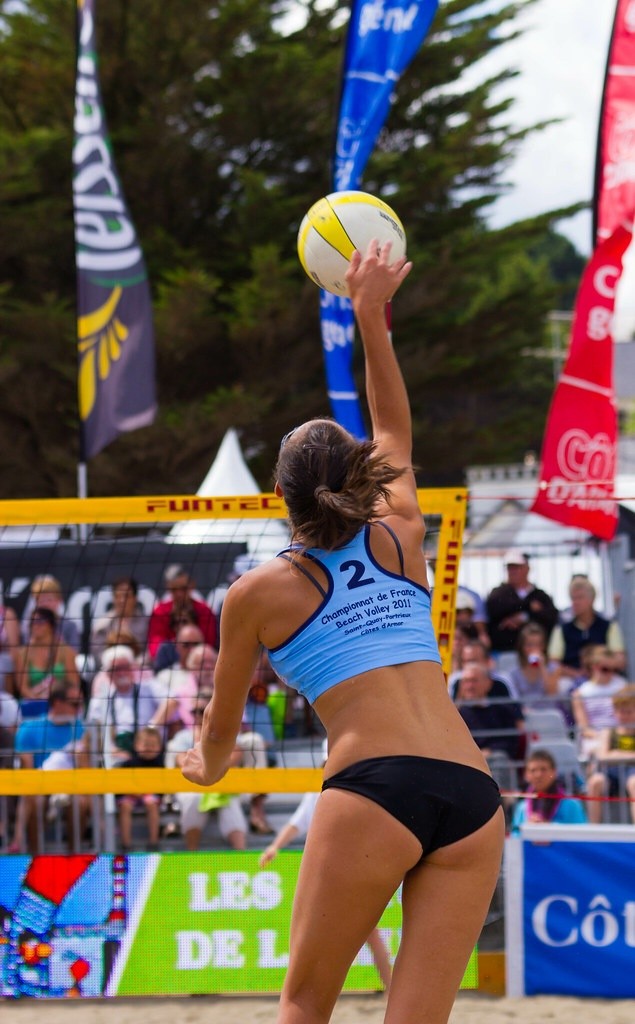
[167,240,505,1024]
[1,549,635,855]
[258,736,391,998]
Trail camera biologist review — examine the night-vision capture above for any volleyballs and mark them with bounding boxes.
[297,191,406,297]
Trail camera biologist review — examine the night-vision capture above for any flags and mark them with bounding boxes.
[63,2,159,453]
[311,2,439,459]
[527,2,635,536]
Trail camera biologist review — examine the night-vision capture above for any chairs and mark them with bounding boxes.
[0,569,634,833]
